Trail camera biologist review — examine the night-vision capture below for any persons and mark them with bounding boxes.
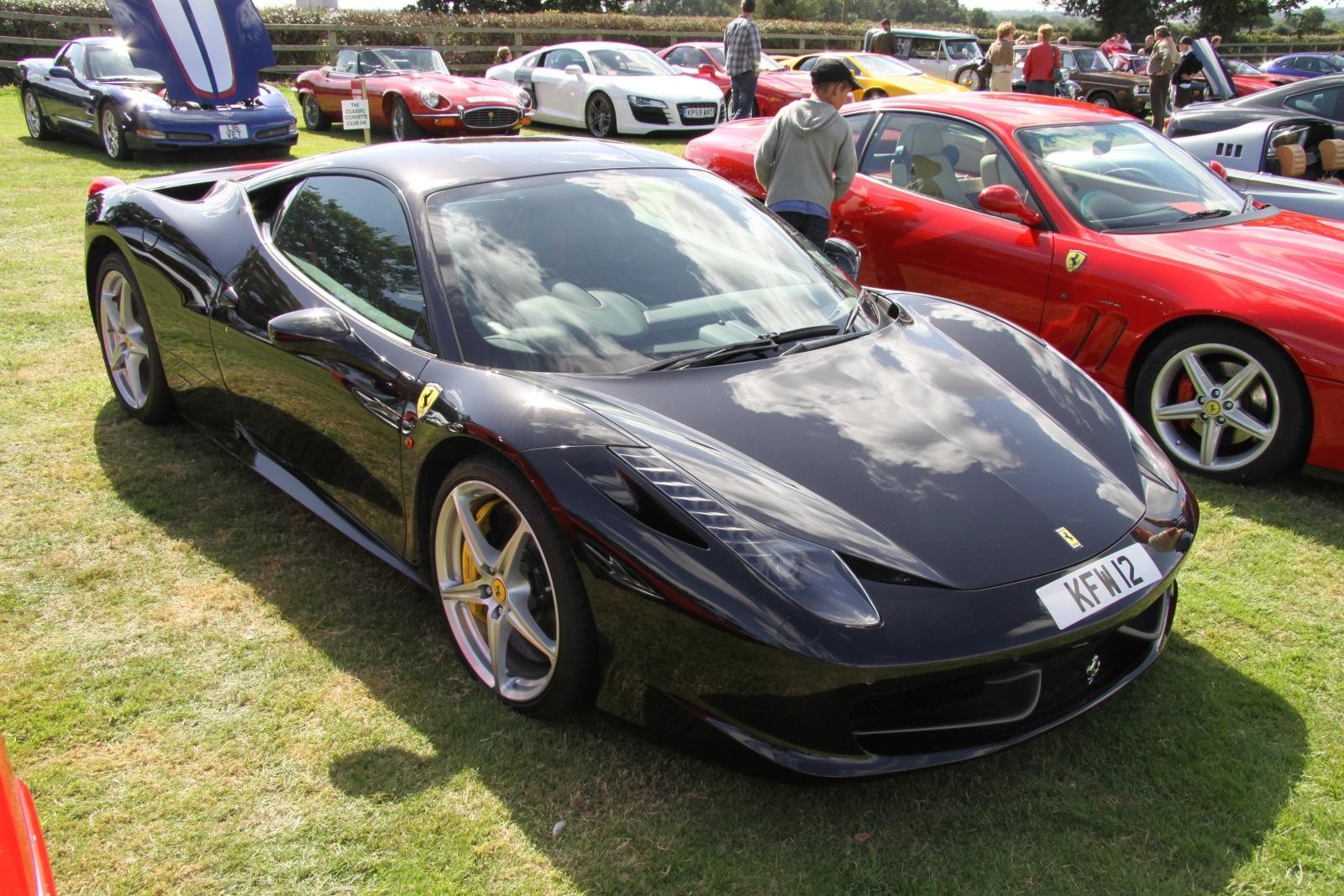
[1023,22,1061,96]
[1146,25,1182,131]
[497,47,512,61]
[869,19,897,56]
[1173,36,1201,110]
[1015,33,1028,57]
[988,21,1016,93]
[1211,35,1222,48]
[1137,35,1155,57]
[753,58,863,251]
[1091,32,1131,71]
[723,0,761,119]
[1058,36,1068,45]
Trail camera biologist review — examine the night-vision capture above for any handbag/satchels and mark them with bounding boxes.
[1053,67,1063,82]
[976,60,991,80]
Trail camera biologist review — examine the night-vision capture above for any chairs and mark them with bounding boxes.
[1310,61,1321,72]
[1311,93,1335,111]
[890,121,975,211]
[980,133,1107,231]
[1276,144,1307,178]
[1316,138,1344,187]
[1301,125,1335,156]
[596,53,621,70]
[701,48,723,64]
[556,55,573,70]
[348,59,411,74]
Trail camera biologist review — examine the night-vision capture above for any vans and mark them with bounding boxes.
[864,28,988,91]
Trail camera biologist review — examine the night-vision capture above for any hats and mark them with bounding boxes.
[810,57,864,92]
[879,18,891,26]
[1177,36,1193,46]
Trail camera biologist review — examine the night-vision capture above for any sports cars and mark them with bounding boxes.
[977,33,1344,228]
[684,91,1344,489]
[617,41,855,118]
[82,132,1201,786]
[290,45,536,144]
[485,40,727,140]
[13,0,303,163]
[766,50,972,103]
